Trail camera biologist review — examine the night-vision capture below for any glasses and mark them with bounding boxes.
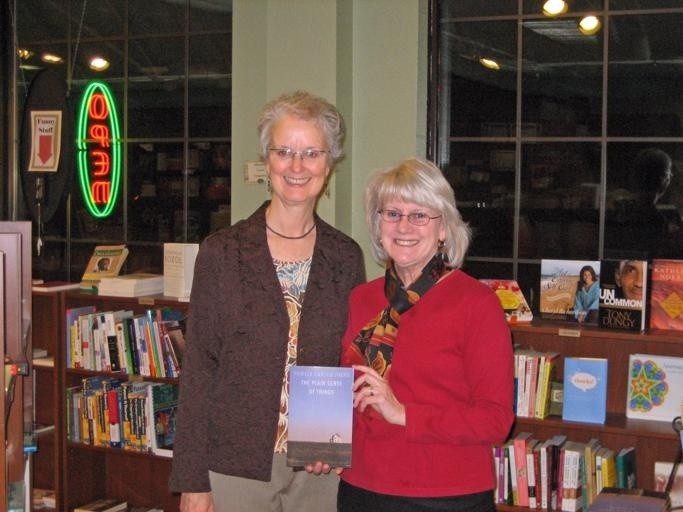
[266,147,331,160]
[377,206,442,226]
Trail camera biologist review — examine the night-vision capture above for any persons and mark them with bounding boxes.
[573,265,600,323]
[169,91,366,512]
[615,147,683,253]
[614,261,642,301]
[98,258,110,272]
[307,157,515,512]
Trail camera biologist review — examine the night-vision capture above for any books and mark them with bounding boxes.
[650,259,683,332]
[66,305,189,379]
[76,495,177,512]
[513,354,561,420]
[288,365,354,471]
[163,244,199,297]
[480,278,534,325]
[66,376,178,457]
[32,488,58,509]
[493,432,683,512]
[81,245,129,285]
[32,347,55,368]
[540,260,601,328]
[600,259,646,333]
[563,356,609,425]
[97,272,164,297]
[32,279,82,293]
[627,352,682,422]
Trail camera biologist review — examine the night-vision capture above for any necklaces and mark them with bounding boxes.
[266,225,316,240]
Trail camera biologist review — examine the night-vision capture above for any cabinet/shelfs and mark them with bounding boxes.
[30,288,62,511]
[493,315,683,512]
[1,220,37,512]
[61,280,190,512]
